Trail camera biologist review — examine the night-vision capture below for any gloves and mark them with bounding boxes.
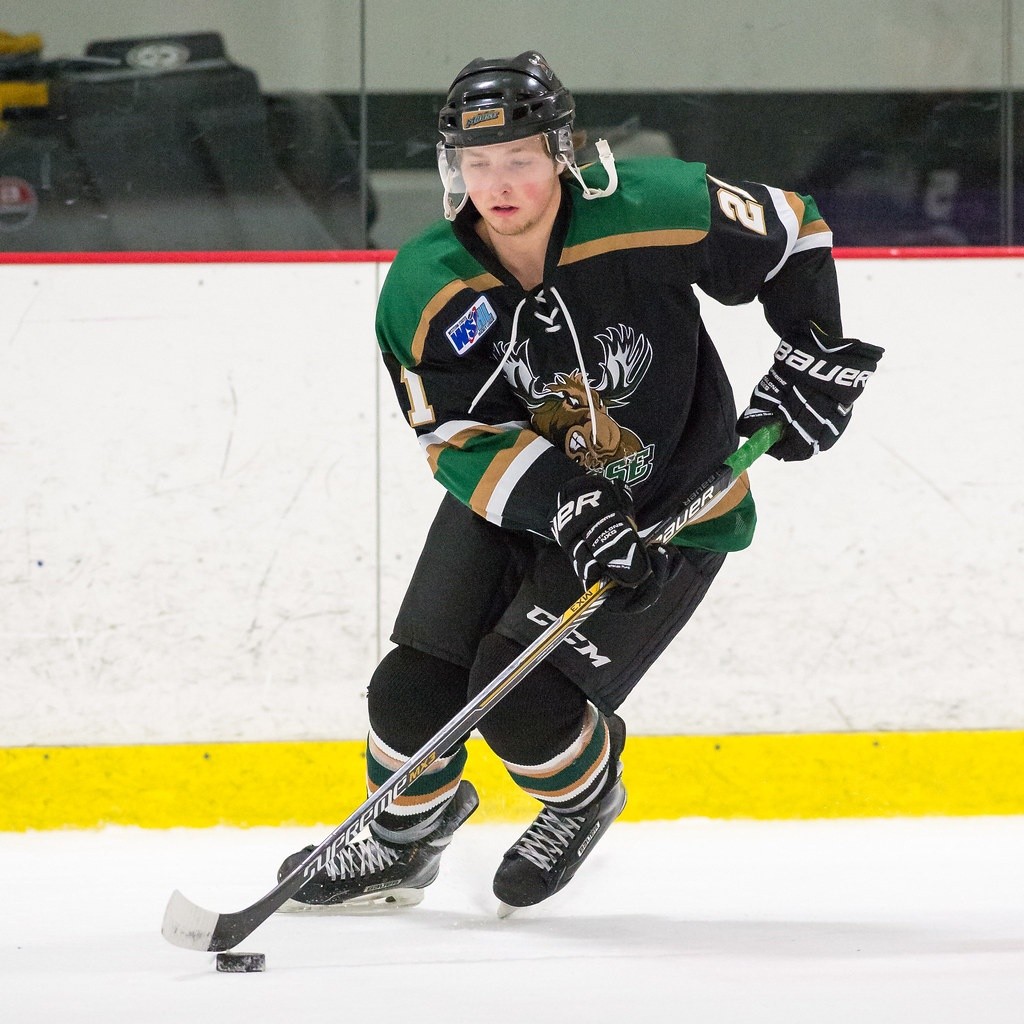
[552,468,684,617]
[735,338,886,460]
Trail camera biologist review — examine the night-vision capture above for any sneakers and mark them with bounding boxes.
[278,780,479,915]
[492,713,627,920]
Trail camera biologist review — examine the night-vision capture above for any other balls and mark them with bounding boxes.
[213,950,267,974]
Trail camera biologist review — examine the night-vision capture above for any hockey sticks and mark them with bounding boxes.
[160,421,782,951]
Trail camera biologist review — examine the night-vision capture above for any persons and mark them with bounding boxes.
[276,49,885,919]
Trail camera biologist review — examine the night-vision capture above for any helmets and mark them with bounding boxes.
[435,50,576,152]
